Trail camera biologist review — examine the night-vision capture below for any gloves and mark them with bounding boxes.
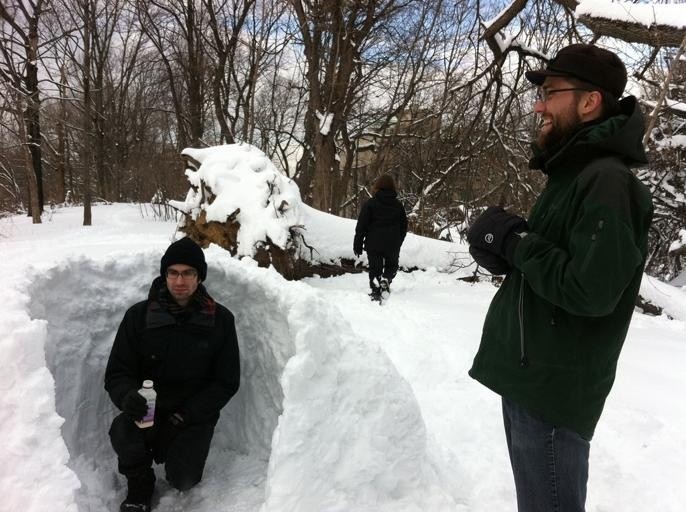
[467,205,528,277]
[152,411,185,464]
[354,248,362,255]
[119,387,148,422]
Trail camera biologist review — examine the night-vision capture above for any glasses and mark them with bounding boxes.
[165,271,197,280]
[536,86,586,101]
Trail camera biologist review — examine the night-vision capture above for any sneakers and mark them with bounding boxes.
[380,277,390,299]
[371,288,380,300]
[121,483,154,512]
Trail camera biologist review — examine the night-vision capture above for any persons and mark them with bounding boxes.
[466,42,653,512]
[101,238,243,511]
[354,174,410,302]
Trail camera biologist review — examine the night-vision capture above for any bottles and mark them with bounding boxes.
[135,380,157,430]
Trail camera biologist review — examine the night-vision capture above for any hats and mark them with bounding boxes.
[160,236,207,281]
[525,44,626,96]
